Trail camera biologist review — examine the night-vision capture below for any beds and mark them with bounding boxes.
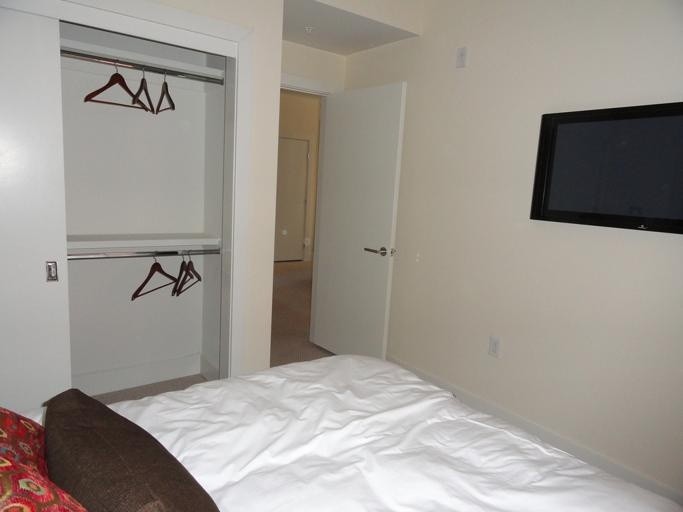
[32,344,682,511]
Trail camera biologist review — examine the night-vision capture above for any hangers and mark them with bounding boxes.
[84,55,176,115]
[131,249,201,300]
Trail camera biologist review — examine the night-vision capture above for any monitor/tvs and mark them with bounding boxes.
[529,101,683,235]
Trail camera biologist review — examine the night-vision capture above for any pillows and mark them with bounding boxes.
[0,388,218,512]
